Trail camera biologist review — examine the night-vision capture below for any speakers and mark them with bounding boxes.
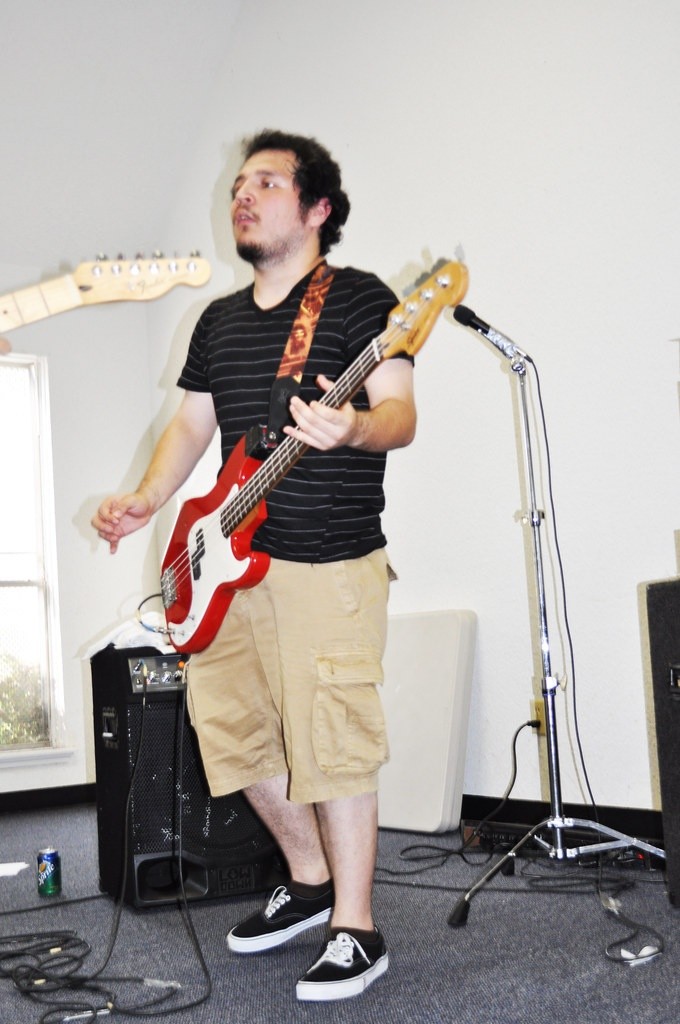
[647,579,680,908]
[90,645,292,908]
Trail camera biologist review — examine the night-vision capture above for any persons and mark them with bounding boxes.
[91,132,417,1001]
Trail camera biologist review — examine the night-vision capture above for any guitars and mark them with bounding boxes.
[157,259,477,659]
[0,247,211,339]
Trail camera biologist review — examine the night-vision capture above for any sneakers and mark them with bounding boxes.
[227,880,338,955]
[296,917,389,1003]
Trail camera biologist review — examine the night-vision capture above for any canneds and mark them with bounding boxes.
[37,848,62,896]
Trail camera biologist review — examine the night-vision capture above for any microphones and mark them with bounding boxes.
[454,303,532,363]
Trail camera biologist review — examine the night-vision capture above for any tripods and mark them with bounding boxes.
[448,354,666,931]
